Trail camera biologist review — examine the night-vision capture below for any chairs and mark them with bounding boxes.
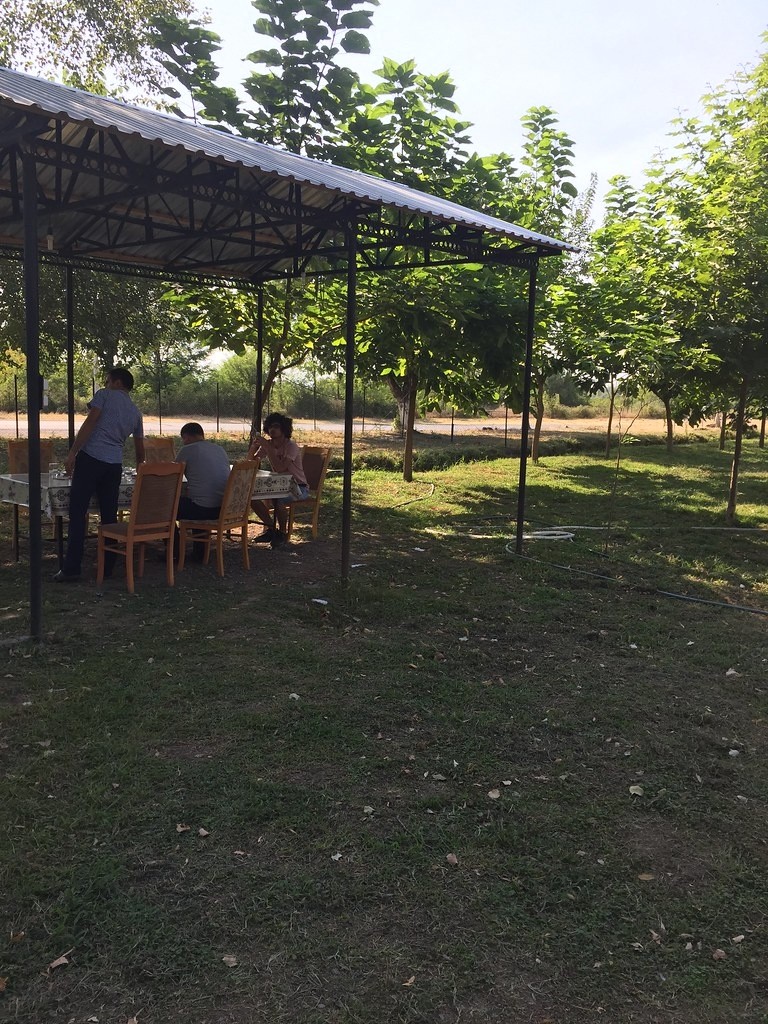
[97,460,186,594]
[143,436,177,462]
[177,459,262,577]
[8,438,97,568]
[262,444,333,543]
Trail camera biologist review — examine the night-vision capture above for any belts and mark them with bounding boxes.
[298,484,306,487]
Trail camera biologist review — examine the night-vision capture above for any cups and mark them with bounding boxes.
[125,467,133,481]
[48,463,61,479]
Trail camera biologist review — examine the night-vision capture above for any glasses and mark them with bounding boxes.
[267,423,281,429]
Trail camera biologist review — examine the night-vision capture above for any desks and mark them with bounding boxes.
[0,465,302,570]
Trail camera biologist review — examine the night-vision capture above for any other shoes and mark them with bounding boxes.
[252,529,278,543]
[189,549,203,563]
[271,532,286,546]
[52,570,80,581]
[104,569,113,579]
[173,541,179,559]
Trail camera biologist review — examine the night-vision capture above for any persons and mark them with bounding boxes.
[47,368,147,583]
[158,422,230,567]
[246,413,309,548]
